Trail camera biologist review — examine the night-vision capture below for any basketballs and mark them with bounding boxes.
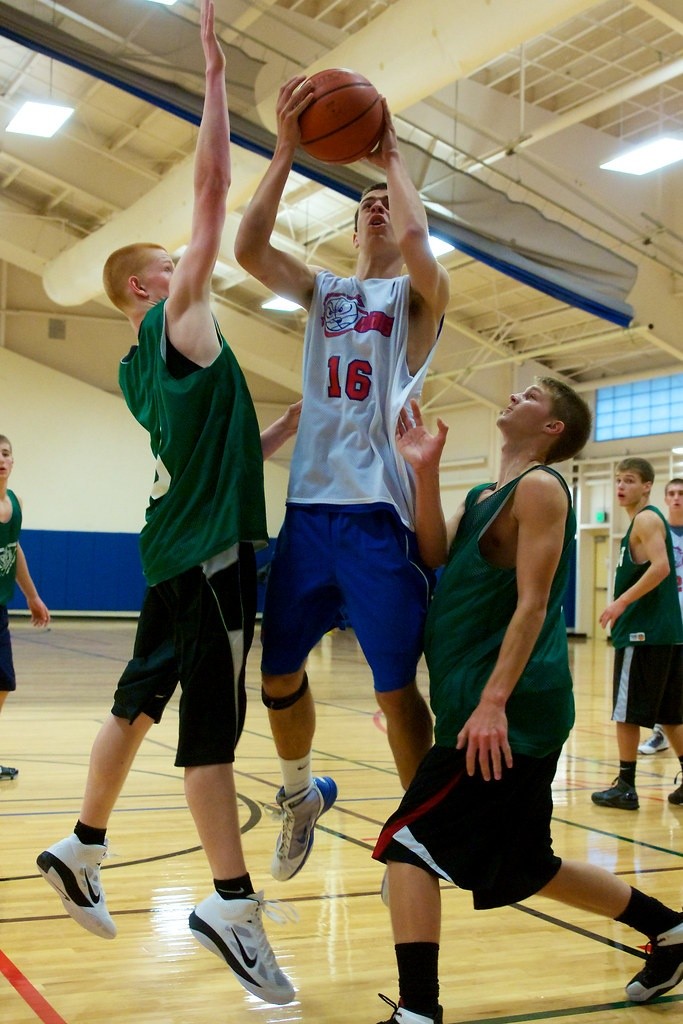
[291,67,385,166]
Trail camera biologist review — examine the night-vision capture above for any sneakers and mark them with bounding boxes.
[668,771,683,805]
[377,993,445,1024]
[591,777,640,810]
[626,907,683,1004]
[638,723,671,754]
[36,832,118,939]
[270,775,339,881]
[189,890,298,1005]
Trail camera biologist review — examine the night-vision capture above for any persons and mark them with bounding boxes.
[591,458,683,810]
[0,433,49,780]
[235,73,449,907]
[370,379,682,1024]
[36,0,296,1005]
[664,478,683,622]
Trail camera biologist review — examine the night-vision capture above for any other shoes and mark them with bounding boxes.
[381,867,391,907]
[0,766,19,780]
